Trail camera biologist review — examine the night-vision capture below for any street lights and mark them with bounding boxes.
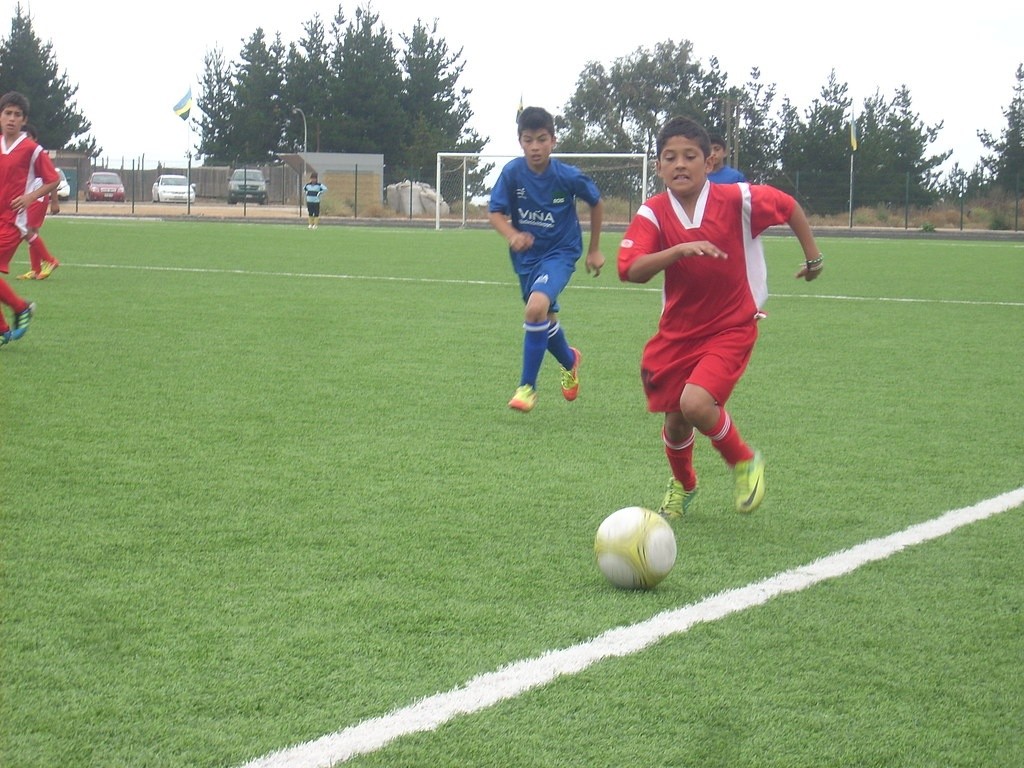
[294,108,308,183]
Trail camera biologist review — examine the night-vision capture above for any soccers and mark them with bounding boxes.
[595,506,678,590]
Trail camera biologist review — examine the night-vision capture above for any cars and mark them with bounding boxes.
[152,175,198,204]
[86,171,125,200]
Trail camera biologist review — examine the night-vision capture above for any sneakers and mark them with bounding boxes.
[12,303,33,340]
[657,476,700,523]
[561,347,582,401]
[37,260,58,279]
[509,386,537,411]
[16,270,39,279]
[735,448,767,514]
[0,331,11,348]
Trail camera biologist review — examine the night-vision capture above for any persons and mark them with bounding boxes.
[0,89,61,346]
[301,172,328,229]
[616,112,825,523]
[16,121,59,282]
[485,104,606,412]
[703,131,749,189]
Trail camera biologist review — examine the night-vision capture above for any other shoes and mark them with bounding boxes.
[308,224,318,229]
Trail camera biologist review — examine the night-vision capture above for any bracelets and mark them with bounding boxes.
[805,260,825,273]
[805,256,823,265]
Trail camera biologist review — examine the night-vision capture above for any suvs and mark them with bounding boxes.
[226,169,270,204]
[47,167,72,200]
